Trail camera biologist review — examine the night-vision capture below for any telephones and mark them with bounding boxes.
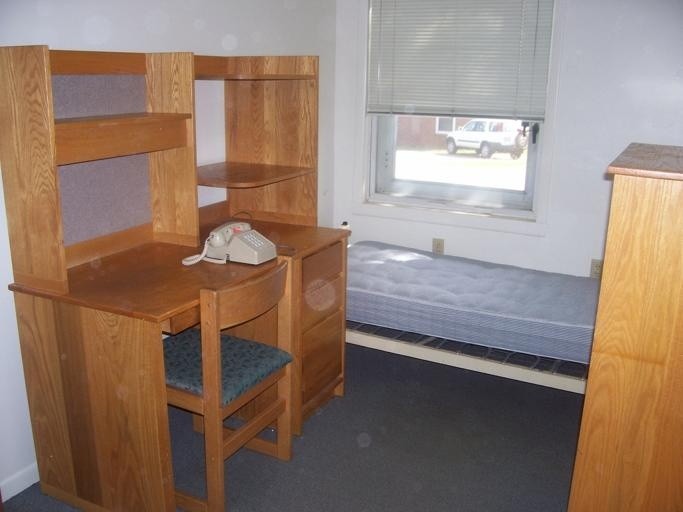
[206,222,277,265]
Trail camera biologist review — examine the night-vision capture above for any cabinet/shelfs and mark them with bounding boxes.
[1,43,319,294]
[566,142,683,512]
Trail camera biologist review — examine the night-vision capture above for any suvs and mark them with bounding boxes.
[447,118,528,158]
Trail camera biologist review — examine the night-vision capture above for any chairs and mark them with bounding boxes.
[162,255,295,512]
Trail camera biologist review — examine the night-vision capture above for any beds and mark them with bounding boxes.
[345,240,601,394]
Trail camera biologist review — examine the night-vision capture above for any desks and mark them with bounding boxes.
[10,217,352,512]
